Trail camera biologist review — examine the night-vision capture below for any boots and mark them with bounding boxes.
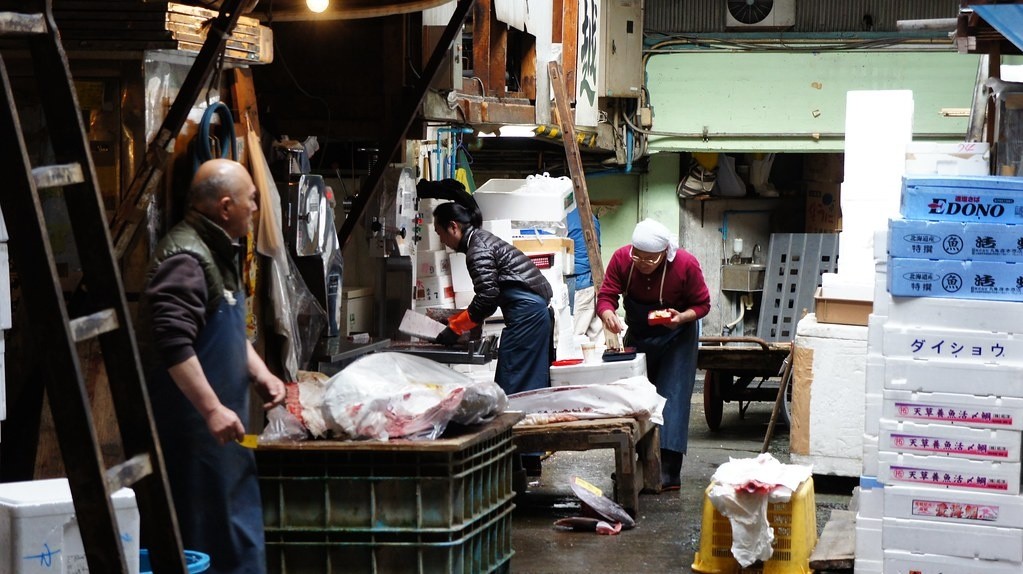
[641,448,682,495]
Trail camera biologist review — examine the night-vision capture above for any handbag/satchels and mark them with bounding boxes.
[674,158,718,199]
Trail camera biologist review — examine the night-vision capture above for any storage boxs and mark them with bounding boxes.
[688,84,1023,573]
[410,167,653,385]
[253,429,519,574]
[0,476,143,574]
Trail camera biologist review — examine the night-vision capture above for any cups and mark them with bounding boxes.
[580,343,596,365]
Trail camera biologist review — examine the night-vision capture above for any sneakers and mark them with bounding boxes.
[522,455,542,474]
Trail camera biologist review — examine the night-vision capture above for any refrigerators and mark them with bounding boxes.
[340,285,375,337]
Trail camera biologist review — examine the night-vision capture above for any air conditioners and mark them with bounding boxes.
[724,0,798,29]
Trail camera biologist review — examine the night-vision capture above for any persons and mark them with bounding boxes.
[434,202,556,480]
[594,216,711,495]
[137,157,287,574]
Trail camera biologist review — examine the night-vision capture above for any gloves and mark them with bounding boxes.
[427,308,482,349]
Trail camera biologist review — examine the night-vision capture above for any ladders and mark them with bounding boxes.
[0,0,194,572]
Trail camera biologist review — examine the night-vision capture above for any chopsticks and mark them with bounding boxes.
[615,313,625,351]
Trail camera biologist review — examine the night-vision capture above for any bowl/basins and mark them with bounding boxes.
[602,347,637,362]
[648,309,672,326]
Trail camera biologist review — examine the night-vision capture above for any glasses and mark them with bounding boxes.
[628,246,663,266]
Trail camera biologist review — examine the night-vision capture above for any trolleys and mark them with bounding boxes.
[696,337,794,434]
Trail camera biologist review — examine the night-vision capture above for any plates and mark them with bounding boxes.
[552,359,584,365]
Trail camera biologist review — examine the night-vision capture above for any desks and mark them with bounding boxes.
[512,385,666,519]
